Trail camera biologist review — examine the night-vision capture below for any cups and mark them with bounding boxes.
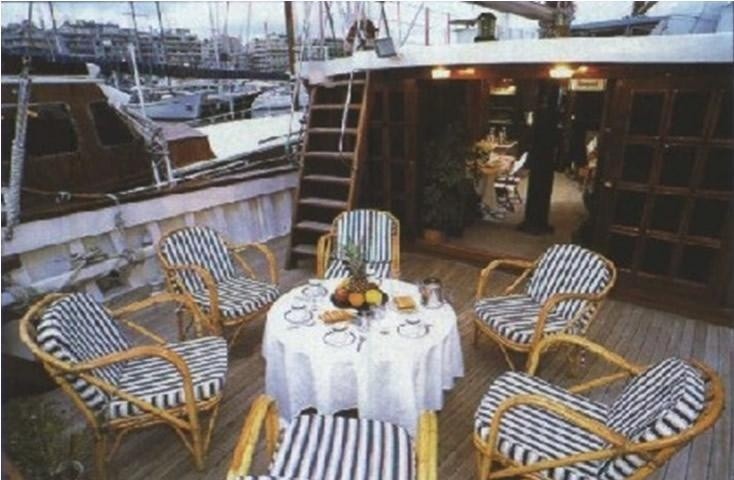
[308,280,320,292]
[292,305,305,319]
[406,319,420,331]
[333,326,347,339]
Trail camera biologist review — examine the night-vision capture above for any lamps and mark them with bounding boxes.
[432,66,450,78]
[489,77,516,95]
[550,65,575,78]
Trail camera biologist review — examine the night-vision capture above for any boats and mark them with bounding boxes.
[1,60,306,222]
[148,89,262,112]
[126,91,206,118]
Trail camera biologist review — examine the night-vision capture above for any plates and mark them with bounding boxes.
[331,291,388,310]
[302,287,326,296]
[397,324,428,337]
[323,332,355,345]
[285,310,311,321]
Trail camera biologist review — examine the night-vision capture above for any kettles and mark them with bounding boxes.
[418,277,447,309]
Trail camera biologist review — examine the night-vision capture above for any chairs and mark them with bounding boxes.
[19,292,229,480]
[316,208,401,278]
[473,358,724,479]
[226,392,438,479]
[472,243,617,383]
[153,225,279,353]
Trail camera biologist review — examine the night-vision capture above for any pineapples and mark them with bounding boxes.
[342,237,369,292]
